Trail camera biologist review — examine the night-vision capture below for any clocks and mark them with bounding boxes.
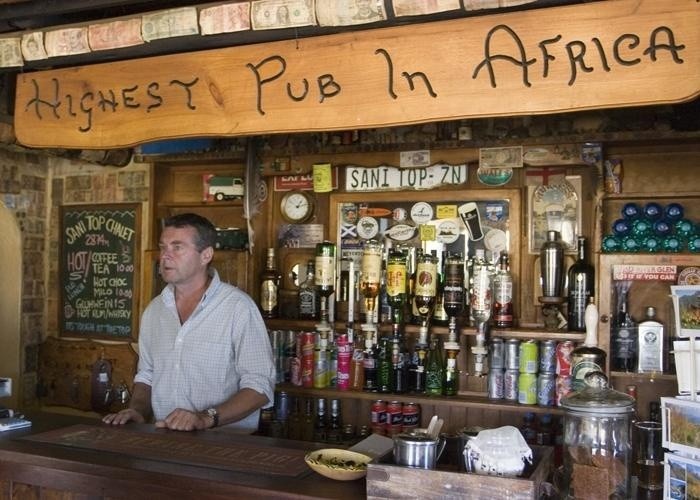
[280,188,316,223]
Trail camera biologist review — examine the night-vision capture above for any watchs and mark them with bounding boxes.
[203,408,218,428]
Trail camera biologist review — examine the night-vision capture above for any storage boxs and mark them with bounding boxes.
[366,430,556,500]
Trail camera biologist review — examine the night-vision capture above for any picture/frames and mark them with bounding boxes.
[527,174,582,255]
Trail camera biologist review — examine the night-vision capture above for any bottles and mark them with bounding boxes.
[613,284,663,372]
[601,202,700,253]
[288,396,342,445]
[541,229,564,297]
[258,245,282,319]
[627,384,639,400]
[567,235,594,330]
[360,240,515,340]
[647,400,660,421]
[298,241,339,324]
[351,341,464,396]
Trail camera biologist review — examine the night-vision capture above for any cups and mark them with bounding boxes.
[459,202,486,241]
[395,435,447,471]
[636,422,664,490]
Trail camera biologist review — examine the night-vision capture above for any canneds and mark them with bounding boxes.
[368,400,421,439]
[267,329,352,389]
[486,339,574,406]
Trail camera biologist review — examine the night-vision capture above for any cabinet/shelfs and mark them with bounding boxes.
[246,311,598,420]
[593,188,699,424]
[135,152,254,311]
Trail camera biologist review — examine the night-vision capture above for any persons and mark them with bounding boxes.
[101,213,277,431]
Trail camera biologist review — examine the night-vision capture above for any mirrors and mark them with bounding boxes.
[326,187,522,318]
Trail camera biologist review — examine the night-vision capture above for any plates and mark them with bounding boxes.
[304,448,372,483]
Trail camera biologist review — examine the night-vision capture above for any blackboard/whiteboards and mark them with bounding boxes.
[58,202,142,343]
